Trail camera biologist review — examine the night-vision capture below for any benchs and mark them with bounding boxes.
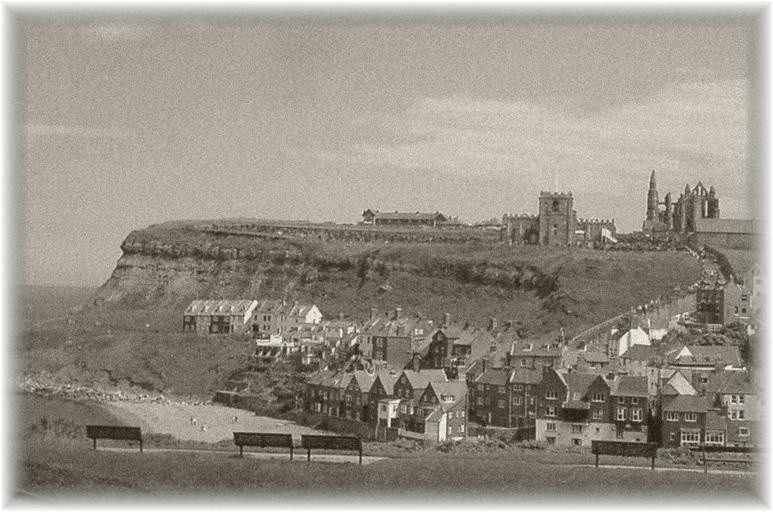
[590,439,658,470]
[691,445,755,473]
[233,432,363,466]
[85,424,143,453]
